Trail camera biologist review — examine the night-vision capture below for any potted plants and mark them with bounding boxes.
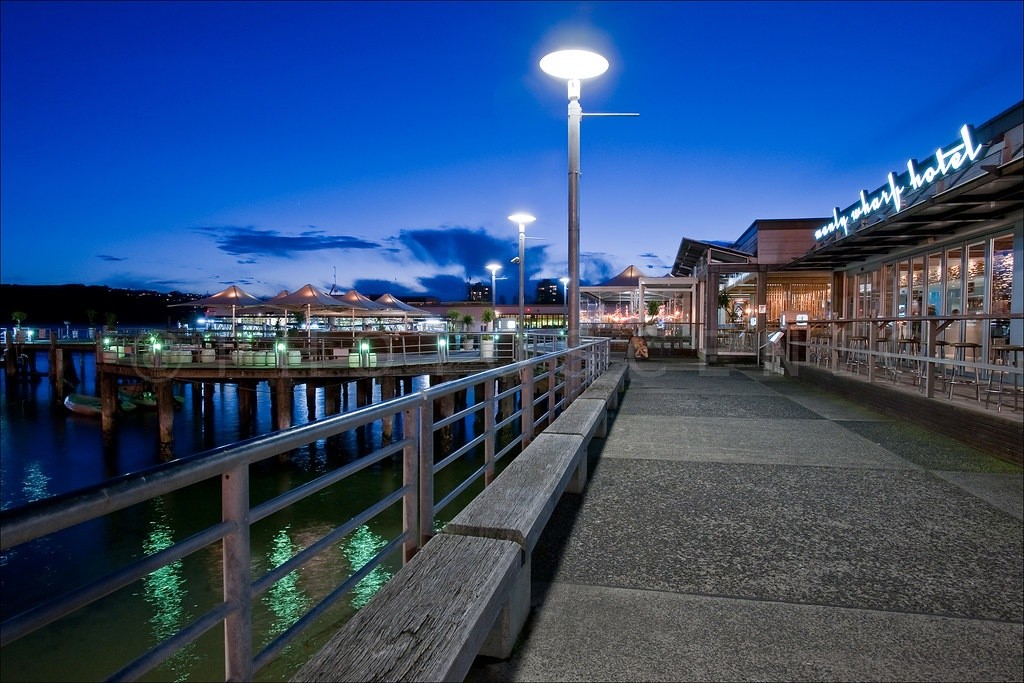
[462,314,474,350]
[449,309,461,350]
[637,299,664,337]
[480,308,497,361]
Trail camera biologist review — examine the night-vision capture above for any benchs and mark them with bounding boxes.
[596,362,630,391]
[542,399,608,451]
[576,380,618,410]
[443,432,587,660]
[288,532,522,683]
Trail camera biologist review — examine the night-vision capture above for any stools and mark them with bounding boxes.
[810,335,820,365]
[817,335,840,369]
[983,345,1024,413]
[893,339,920,387]
[947,343,987,402]
[919,341,948,395]
[845,336,869,376]
[866,338,891,384]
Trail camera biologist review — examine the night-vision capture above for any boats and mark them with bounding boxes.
[63,384,187,418]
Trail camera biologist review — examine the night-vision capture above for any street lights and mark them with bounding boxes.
[483,262,502,312]
[542,49,613,407]
[559,278,570,307]
[507,208,536,359]
